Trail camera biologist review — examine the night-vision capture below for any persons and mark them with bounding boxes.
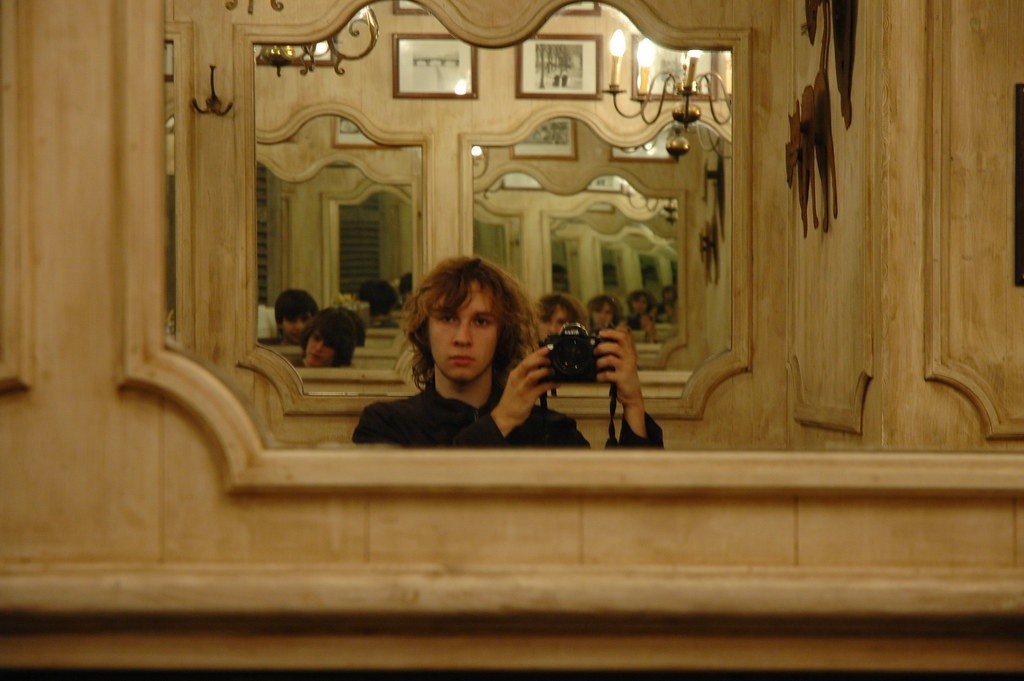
[352,255,666,450]
[586,295,629,335]
[627,289,657,329]
[400,272,412,303]
[272,289,318,346]
[357,280,400,328]
[538,292,586,335]
[657,286,678,322]
[292,307,363,367]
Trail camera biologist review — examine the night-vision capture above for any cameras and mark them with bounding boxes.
[537,302,667,382]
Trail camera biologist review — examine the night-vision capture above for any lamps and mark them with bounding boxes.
[604,30,732,164]
[257,5,379,76]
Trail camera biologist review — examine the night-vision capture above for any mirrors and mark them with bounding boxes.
[253,105,432,384]
[476,197,525,286]
[257,151,423,330]
[231,0,752,421]
[542,197,681,347]
[111,0,1024,504]
[454,106,736,387]
[159,0,195,359]
[318,180,418,330]
[474,164,689,372]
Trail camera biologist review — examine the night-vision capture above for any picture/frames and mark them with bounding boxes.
[629,34,718,102]
[514,36,603,100]
[329,115,403,150]
[607,123,677,165]
[390,31,479,102]
[554,0,601,16]
[508,119,578,161]
[392,0,429,15]
[254,40,339,67]
